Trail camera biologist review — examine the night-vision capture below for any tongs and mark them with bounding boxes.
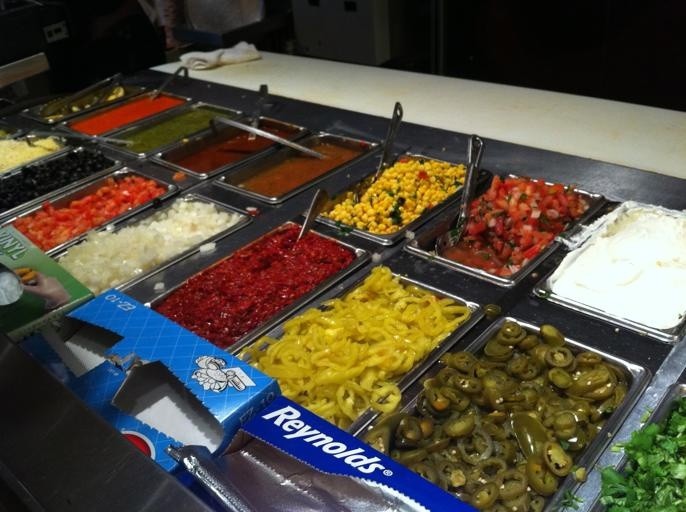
[39,73,123,120]
